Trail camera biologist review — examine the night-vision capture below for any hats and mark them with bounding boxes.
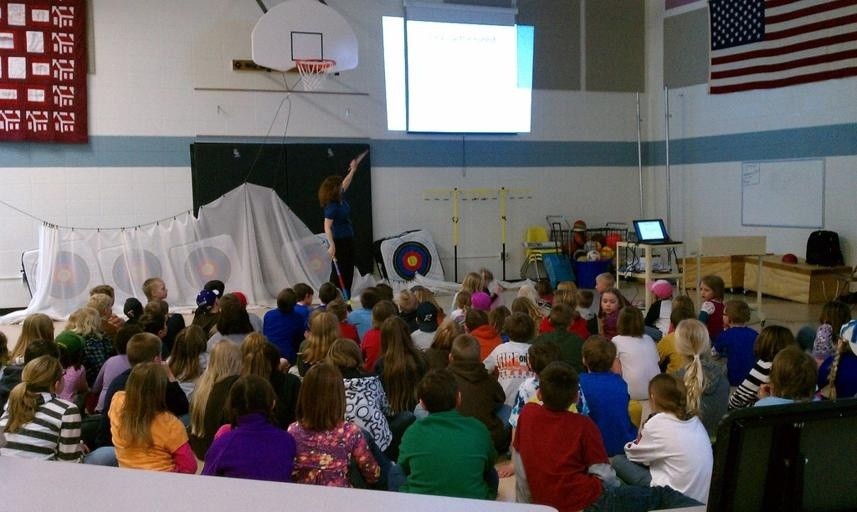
[195,289,220,310]
[415,301,440,332]
[651,279,673,300]
[123,298,144,319]
[471,292,490,311]
[203,280,226,297]
[231,290,248,307]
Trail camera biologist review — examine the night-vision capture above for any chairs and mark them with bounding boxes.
[518,226,563,283]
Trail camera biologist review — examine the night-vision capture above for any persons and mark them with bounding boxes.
[317,158,357,311]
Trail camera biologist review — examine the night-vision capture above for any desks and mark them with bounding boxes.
[741,253,853,305]
[671,252,773,294]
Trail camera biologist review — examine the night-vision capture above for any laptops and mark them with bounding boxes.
[634,218,682,245]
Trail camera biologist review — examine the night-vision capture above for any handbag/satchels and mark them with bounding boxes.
[806,230,844,266]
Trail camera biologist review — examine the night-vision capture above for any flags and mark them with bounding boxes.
[705,0,857,95]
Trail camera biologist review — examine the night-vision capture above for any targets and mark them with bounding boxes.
[169,233,245,308]
[281,231,340,299]
[96,234,182,315]
[372,230,446,290]
[22,239,107,320]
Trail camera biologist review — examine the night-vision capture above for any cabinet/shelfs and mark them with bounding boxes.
[615,240,687,316]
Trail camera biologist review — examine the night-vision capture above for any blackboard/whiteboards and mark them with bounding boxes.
[741,158,826,229]
[189,142,375,277]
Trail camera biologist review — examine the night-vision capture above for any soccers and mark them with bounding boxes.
[563,220,622,262]
